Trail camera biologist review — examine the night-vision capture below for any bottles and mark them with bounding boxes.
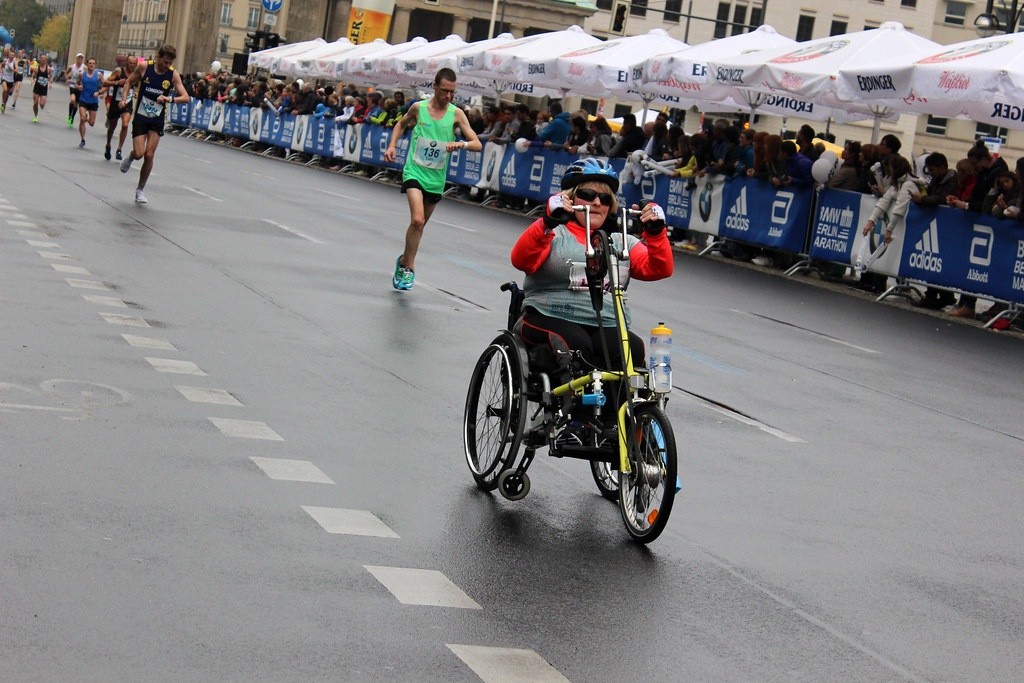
[649,322,673,393]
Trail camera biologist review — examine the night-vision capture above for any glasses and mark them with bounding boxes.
[970,159,980,167]
[574,188,613,206]
[437,84,458,96]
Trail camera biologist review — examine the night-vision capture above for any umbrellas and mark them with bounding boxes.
[249,22,1024,150]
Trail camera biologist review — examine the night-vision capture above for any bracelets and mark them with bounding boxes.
[461,139,469,150]
[170,95,174,103]
[964,201,968,211]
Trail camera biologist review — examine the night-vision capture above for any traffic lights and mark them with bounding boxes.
[245,30,260,53]
[264,32,288,49]
[608,0,632,37]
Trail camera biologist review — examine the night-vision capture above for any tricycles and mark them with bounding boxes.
[463,203,678,544]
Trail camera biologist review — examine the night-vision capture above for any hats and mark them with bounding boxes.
[76,52,84,58]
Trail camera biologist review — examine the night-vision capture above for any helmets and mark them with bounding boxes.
[561,158,620,194]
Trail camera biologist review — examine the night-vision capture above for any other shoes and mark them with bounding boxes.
[848,275,886,293]
[710,244,747,260]
[681,243,706,250]
[105,145,122,160]
[948,306,975,316]
[67,116,74,128]
[12,102,16,107]
[135,189,147,203]
[976,305,1009,321]
[910,293,957,310]
[1,105,6,112]
[81,140,86,146]
[674,239,689,248]
[120,153,135,173]
[751,255,774,267]
[34,118,38,122]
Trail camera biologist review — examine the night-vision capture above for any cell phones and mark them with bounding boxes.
[781,174,788,182]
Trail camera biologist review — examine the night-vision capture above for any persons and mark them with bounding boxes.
[510,157,673,437]
[0,45,1024,334]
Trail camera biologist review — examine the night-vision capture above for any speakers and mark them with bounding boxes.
[231,52,249,75]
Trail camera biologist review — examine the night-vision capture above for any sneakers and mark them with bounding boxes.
[392,255,415,290]
